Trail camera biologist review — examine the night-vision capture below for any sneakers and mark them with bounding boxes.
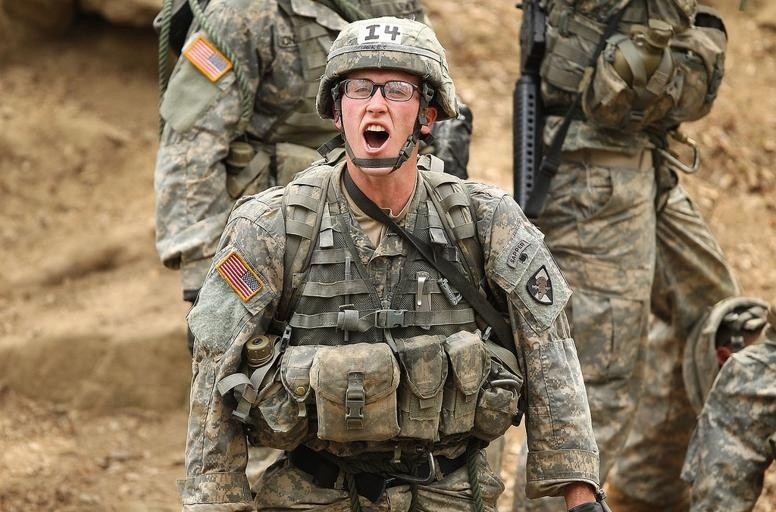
[605,482,689,512]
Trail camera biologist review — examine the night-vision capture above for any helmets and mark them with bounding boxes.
[682,296,769,415]
[316,16,458,121]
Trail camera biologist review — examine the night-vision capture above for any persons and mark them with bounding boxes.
[508,1,740,512]
[182,15,611,512]
[674,294,776,512]
[150,2,474,327]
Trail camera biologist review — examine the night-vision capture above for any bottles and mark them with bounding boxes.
[244,334,306,434]
[221,139,256,178]
[590,16,672,130]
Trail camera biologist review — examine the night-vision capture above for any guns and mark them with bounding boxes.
[512,1,546,216]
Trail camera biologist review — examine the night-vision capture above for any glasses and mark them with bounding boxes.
[339,78,423,102]
